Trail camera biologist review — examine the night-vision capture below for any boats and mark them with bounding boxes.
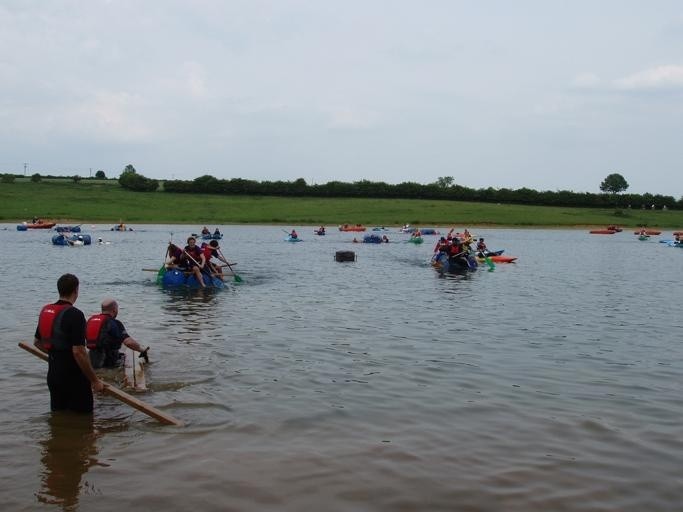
[589,228,682,248]
[200,234,221,239]
[316,225,439,244]
[17,222,133,245]
[283,232,300,242]
[430,231,517,274]
[156,261,224,294]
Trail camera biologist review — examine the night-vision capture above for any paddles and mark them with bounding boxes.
[471,240,495,269]
[217,248,242,281]
[158,245,169,275]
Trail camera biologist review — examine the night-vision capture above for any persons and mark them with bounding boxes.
[162,242,186,270]
[84,298,144,369]
[34,273,110,417]
[201,226,209,234]
[203,240,225,277]
[639,226,645,237]
[32,216,38,224]
[181,236,206,289]
[288,229,297,238]
[38,418,111,511]
[411,229,421,238]
[213,228,220,235]
[119,222,124,228]
[200,241,221,273]
[432,227,486,257]
[318,225,325,232]
